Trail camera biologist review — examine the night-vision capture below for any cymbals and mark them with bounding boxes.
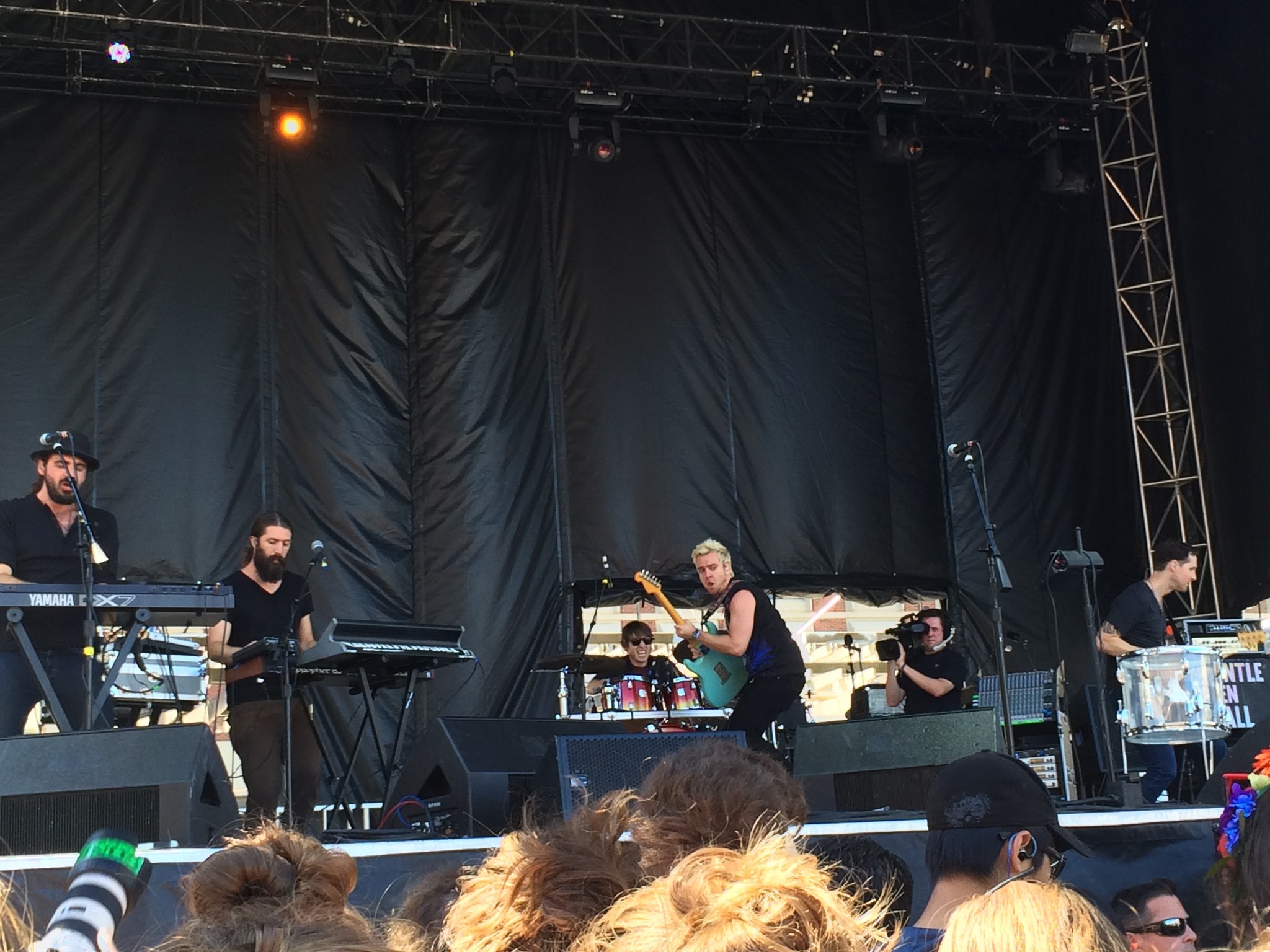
[533,653,626,674]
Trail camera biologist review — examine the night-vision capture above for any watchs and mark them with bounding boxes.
[692,628,701,640]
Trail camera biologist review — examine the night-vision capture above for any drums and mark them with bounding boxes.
[599,674,656,713]
[654,676,705,710]
[1114,642,1233,744]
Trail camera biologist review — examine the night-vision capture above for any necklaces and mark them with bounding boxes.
[1146,578,1155,592]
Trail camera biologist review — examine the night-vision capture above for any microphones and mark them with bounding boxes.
[880,625,900,634]
[844,633,853,655]
[600,549,614,591]
[947,439,980,460]
[128,634,146,672]
[37,429,72,446]
[310,538,330,572]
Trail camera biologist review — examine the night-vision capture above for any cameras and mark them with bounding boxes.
[27,831,153,952]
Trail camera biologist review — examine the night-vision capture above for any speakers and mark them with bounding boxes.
[1066,683,1138,776]
[0,723,245,857]
[1198,716,1270,809]
[538,731,747,828]
[383,715,623,842]
[788,707,1010,826]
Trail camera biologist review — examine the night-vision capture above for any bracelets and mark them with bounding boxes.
[900,663,907,670]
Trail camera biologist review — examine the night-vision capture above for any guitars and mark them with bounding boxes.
[631,568,752,708]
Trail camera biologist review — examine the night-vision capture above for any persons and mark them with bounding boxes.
[0,743,1270,952]
[207,512,322,838]
[1,429,122,737]
[1090,542,1200,803]
[675,538,806,761]
[586,621,689,711]
[884,608,969,715]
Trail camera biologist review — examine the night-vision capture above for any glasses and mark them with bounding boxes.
[1128,917,1197,937]
[997,831,1067,880]
[626,637,655,646]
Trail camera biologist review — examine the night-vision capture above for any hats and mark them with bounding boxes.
[927,748,1095,859]
[31,431,100,472]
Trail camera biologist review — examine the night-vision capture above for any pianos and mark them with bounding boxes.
[1,578,236,629]
[270,617,469,680]
